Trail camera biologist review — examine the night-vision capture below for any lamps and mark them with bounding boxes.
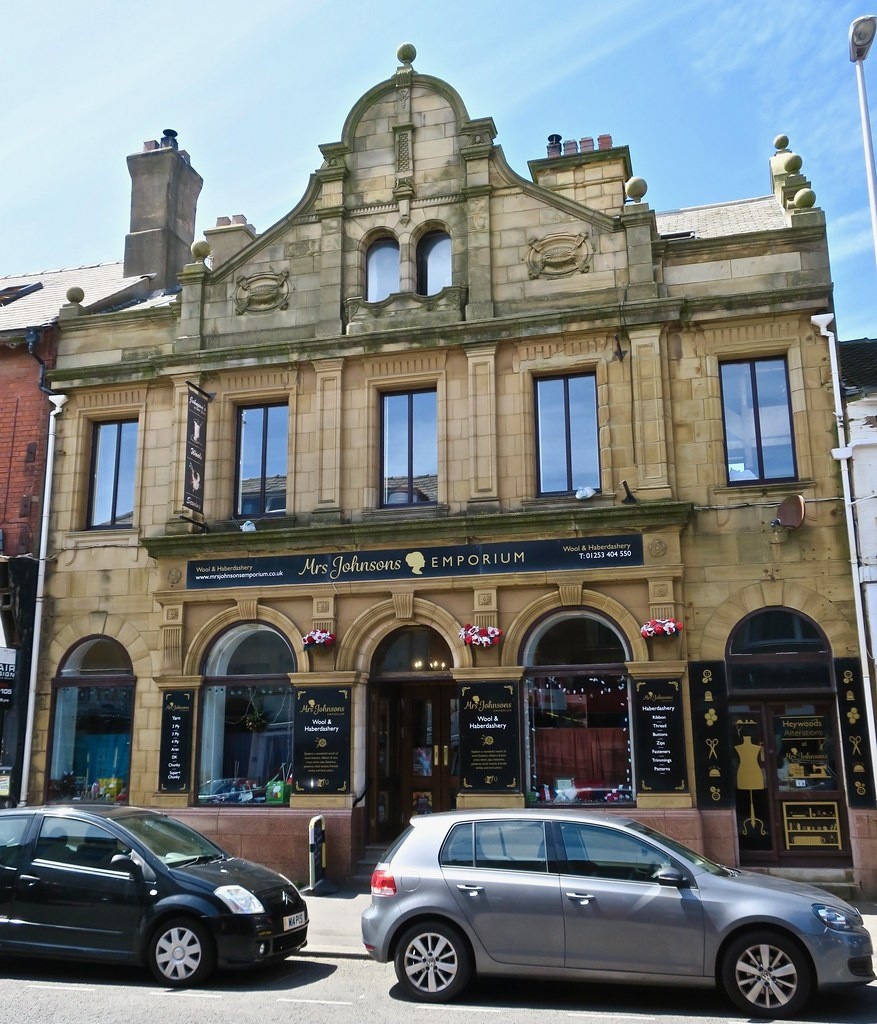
[414,655,448,672]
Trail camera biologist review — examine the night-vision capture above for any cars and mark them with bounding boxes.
[195,779,283,803]
[0,805,317,986]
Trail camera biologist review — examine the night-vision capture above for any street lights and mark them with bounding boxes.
[847,12,877,261]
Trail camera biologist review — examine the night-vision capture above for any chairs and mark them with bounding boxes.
[75,827,119,868]
[451,837,491,868]
[562,828,600,878]
[38,827,80,866]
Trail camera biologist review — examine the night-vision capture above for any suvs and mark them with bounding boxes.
[359,809,874,1020]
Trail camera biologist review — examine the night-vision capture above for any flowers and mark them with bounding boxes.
[639,617,682,640]
[301,629,340,654]
[457,623,504,648]
